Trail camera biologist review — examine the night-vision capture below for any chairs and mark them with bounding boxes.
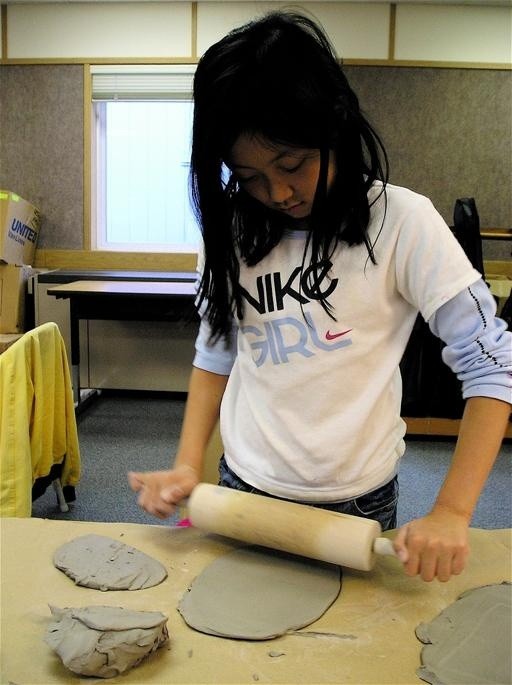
[0,322,70,513]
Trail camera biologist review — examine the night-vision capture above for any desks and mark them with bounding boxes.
[45,278,202,425]
[0,517,511,685]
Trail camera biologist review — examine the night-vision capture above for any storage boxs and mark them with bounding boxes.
[0,266,35,334]
[0,188,41,266]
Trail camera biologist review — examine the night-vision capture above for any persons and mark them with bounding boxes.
[123,1,512,585]
